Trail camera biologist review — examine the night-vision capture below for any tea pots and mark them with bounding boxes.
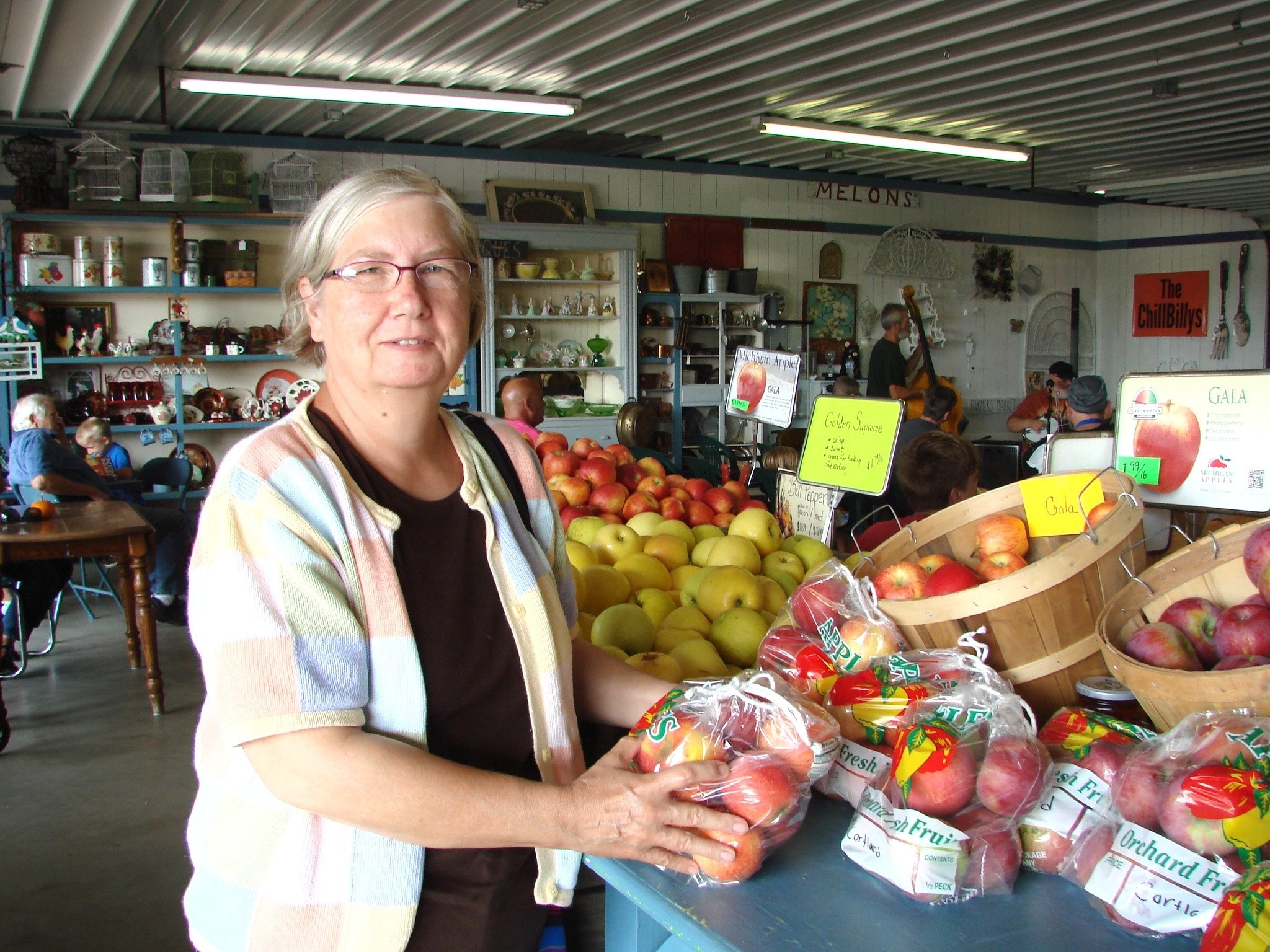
[146,401,177,425]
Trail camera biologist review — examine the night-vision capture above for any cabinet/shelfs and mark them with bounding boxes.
[898,281,946,357]
[1,210,478,513]
[635,291,868,469]
[473,219,638,448]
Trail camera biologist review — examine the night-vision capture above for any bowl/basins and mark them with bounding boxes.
[511,265,540,279]
[588,404,618,415]
[639,373,659,389]
[542,394,584,416]
[576,403,589,413]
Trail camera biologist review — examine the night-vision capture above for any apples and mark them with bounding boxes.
[1124,521,1270,671]
[565,508,873,684]
[873,501,1119,601]
[519,432,768,528]
[759,580,1045,891]
[1133,400,1200,493]
[736,362,766,412]
[636,688,837,884]
[1023,716,1263,933]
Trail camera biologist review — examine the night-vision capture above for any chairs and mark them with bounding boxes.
[0,457,194,680]
[625,427,808,516]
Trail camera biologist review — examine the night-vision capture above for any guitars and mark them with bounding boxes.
[1022,415,1069,448]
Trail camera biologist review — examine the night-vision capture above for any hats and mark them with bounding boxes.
[1067,375,1107,414]
[1049,361,1074,380]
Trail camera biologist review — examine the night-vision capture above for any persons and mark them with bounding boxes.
[1006,361,1077,454]
[864,302,938,428]
[830,378,957,521]
[500,374,547,454]
[7,393,189,627]
[0,499,73,672]
[763,446,870,556]
[179,163,753,950]
[851,428,982,558]
[1021,374,1116,482]
[75,416,141,569]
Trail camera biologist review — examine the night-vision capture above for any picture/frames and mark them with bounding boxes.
[37,300,115,358]
[485,179,596,225]
[60,365,101,402]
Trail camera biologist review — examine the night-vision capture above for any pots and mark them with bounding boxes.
[616,397,654,449]
[682,407,703,446]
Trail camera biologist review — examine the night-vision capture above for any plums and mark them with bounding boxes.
[1,507,42,523]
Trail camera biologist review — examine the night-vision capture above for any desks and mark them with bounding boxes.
[0,499,165,716]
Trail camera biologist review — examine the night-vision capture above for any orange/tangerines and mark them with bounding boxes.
[31,493,54,518]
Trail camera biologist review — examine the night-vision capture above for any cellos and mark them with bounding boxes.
[901,285,963,436]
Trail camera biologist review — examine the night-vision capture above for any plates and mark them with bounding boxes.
[193,388,225,419]
[554,340,582,368]
[219,388,253,419]
[286,378,320,409]
[168,443,211,489]
[527,341,554,367]
[241,369,300,420]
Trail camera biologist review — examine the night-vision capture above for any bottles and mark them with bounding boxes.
[845,355,854,380]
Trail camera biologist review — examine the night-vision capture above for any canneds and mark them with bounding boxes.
[1074,676,1145,727]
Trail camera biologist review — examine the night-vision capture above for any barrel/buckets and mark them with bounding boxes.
[703,267,729,293]
[732,267,758,295]
[672,263,702,294]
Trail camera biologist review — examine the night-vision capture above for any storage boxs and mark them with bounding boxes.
[17,231,73,287]
[225,271,256,287]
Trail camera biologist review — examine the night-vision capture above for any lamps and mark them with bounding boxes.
[749,114,1034,163]
[169,69,582,117]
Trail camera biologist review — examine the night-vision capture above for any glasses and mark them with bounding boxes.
[1053,374,1066,383]
[323,258,479,295]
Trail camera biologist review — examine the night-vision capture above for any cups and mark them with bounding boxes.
[184,239,200,287]
[102,235,126,287]
[158,426,174,444]
[138,428,156,447]
[73,236,100,286]
[142,257,168,287]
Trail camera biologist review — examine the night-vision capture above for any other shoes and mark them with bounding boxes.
[151,595,188,626]
[1,645,22,675]
